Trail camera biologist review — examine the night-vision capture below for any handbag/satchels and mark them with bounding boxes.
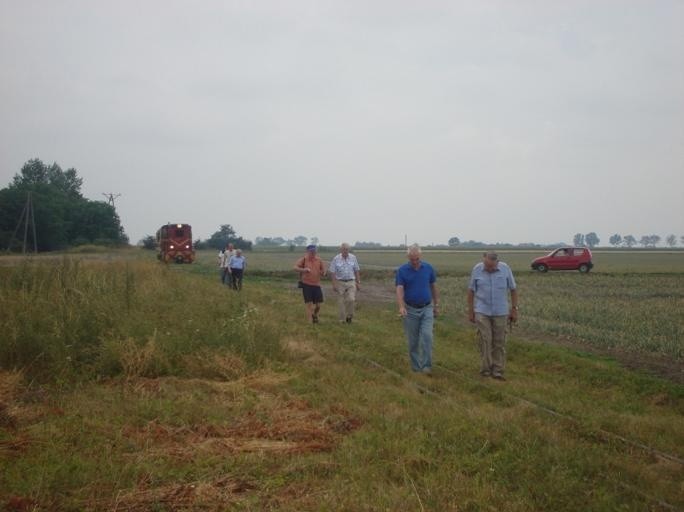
[296,280,304,289]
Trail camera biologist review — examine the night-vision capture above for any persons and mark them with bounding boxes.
[227,249,246,291]
[328,243,361,323]
[466,251,519,377]
[393,246,439,375]
[223,242,236,287]
[218,245,225,283]
[293,244,327,324]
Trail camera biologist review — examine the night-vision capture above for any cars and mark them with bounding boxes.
[530,247,593,273]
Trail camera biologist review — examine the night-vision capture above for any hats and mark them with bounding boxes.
[307,245,317,252]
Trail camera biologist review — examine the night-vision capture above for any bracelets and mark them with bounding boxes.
[433,303,439,307]
[356,280,360,284]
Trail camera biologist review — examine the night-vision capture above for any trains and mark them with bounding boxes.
[157,223,196,264]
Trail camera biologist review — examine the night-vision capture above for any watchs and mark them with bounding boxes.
[512,304,519,310]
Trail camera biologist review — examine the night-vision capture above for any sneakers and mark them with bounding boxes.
[480,369,503,378]
[341,315,352,325]
[422,367,434,377]
[308,315,321,324]
